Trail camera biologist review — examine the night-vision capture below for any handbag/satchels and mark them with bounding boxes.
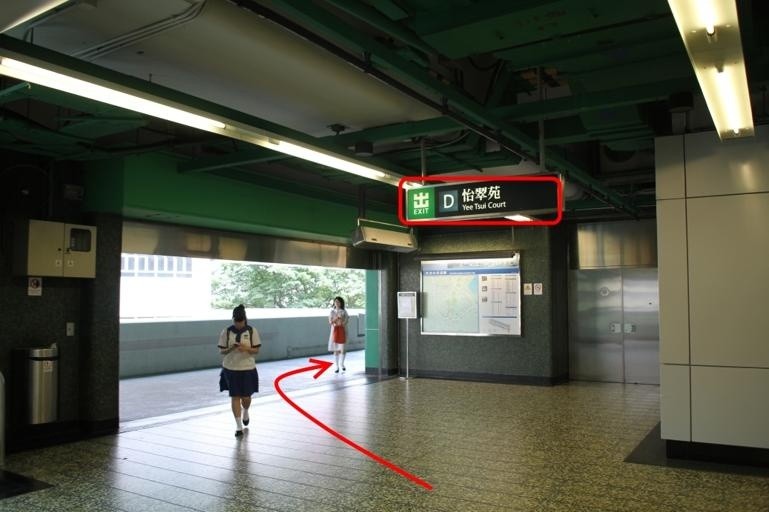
[332,324,346,344]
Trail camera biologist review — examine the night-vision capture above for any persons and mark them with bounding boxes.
[218,303,262,437]
[328,296,349,373]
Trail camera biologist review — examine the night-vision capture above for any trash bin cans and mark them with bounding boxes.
[10,346,59,426]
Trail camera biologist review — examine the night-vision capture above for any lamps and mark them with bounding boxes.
[667,0,757,142]
[0,50,536,235]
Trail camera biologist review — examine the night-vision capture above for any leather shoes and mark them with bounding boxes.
[242,416,250,425]
[234,429,243,436]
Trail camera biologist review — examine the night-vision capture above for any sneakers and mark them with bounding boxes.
[335,368,339,372]
[342,367,346,370]
